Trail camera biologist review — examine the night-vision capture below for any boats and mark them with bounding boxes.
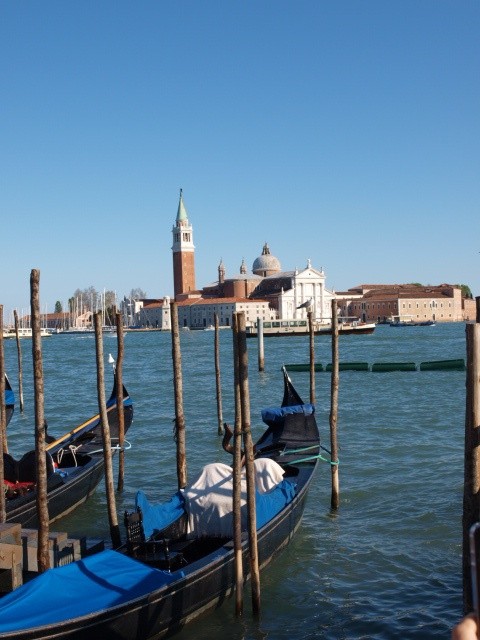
[205,325,216,331]
[112,333,128,337]
[0,367,319,640]
[0,372,132,528]
[390,321,436,326]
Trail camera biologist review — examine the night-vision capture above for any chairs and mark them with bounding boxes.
[124,507,183,572]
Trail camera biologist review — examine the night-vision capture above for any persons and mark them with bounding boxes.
[451,611,480,640]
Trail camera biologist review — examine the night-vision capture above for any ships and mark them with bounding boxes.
[3,303,53,337]
[231,318,376,336]
[55,288,117,332]
[120,296,153,329]
